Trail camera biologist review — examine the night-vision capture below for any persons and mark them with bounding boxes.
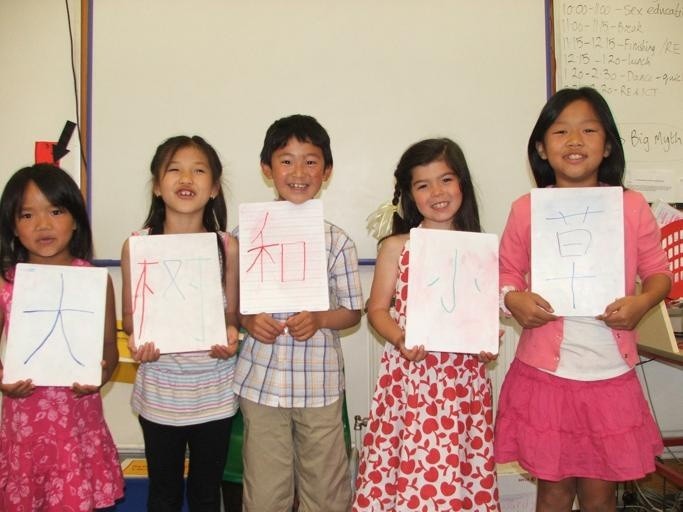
[346,134,506,510]
[228,112,366,511]
[116,131,239,509]
[0,160,124,511]
[495,83,674,511]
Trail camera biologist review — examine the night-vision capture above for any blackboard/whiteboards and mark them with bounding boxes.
[81,0,557,266]
[552,0,683,204]
